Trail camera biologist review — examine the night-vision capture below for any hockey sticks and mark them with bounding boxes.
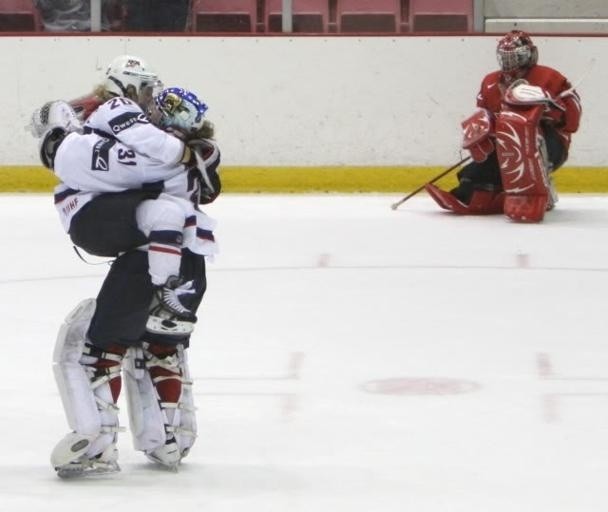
[391,57,598,212]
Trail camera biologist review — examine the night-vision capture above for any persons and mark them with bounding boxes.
[427,29,585,225]
[39,86,224,474]
[49,55,221,335]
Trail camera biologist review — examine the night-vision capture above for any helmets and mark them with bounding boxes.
[104,54,164,106]
[497,29,538,85]
[144,87,210,139]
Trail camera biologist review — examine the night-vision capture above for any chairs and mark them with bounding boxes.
[0,0,473,34]
